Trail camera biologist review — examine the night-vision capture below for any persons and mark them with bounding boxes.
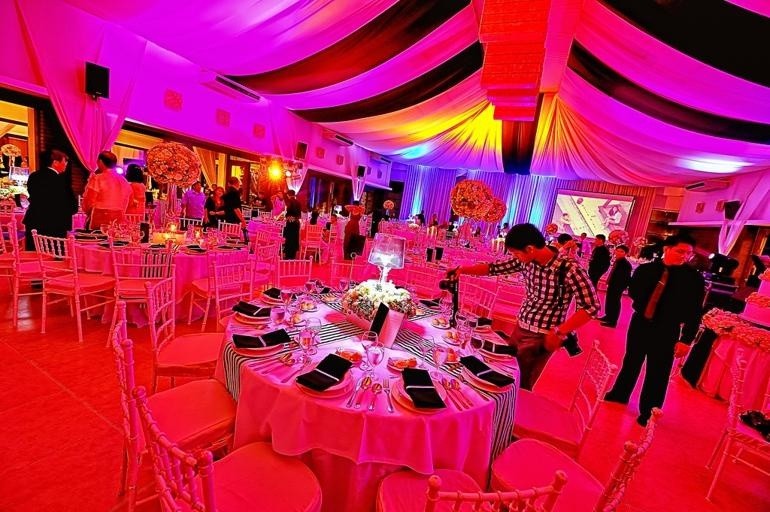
[270,189,303,258]
[497,223,510,237]
[310,203,350,230]
[343,201,365,260]
[179,176,247,230]
[555,233,573,250]
[251,192,267,218]
[22,149,78,288]
[559,213,572,228]
[124,164,147,224]
[416,210,438,227]
[598,245,632,327]
[371,207,400,235]
[581,232,591,258]
[608,206,618,216]
[588,234,611,289]
[604,233,705,427]
[444,224,600,390]
[81,150,131,231]
[603,210,622,228]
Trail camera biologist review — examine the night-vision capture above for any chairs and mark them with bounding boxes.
[0,209,770,511]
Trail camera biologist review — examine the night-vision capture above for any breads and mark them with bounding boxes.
[415,309,425,314]
[446,332,462,341]
[301,302,314,309]
[447,348,455,361]
[293,315,301,323]
[342,350,360,361]
[396,359,417,369]
[433,318,446,325]
[324,294,336,301]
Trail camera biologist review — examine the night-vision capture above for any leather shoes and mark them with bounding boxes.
[598,314,616,327]
[603,393,628,402]
[638,414,648,425]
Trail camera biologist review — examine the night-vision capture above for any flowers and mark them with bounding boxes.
[383,200,394,209]
[1,144,22,157]
[452,180,494,218]
[147,142,201,186]
[483,195,506,223]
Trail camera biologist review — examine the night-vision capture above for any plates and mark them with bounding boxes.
[230,278,520,414]
[71,226,253,255]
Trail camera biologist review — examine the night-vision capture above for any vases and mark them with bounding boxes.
[386,209,389,216]
[9,156,15,167]
[165,182,179,215]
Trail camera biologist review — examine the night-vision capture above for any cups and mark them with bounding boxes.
[405,234,480,268]
[8,164,29,187]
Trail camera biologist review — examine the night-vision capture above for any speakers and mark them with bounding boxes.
[724,201,739,219]
[358,166,365,177]
[295,142,307,160]
[389,181,404,193]
[86,62,109,98]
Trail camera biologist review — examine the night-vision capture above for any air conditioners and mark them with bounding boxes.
[199,71,266,106]
[370,153,392,165]
[683,178,728,192]
[325,132,354,149]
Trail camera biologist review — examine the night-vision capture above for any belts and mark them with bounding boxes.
[518,319,547,335]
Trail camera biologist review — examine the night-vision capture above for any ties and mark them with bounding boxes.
[644,268,669,320]
